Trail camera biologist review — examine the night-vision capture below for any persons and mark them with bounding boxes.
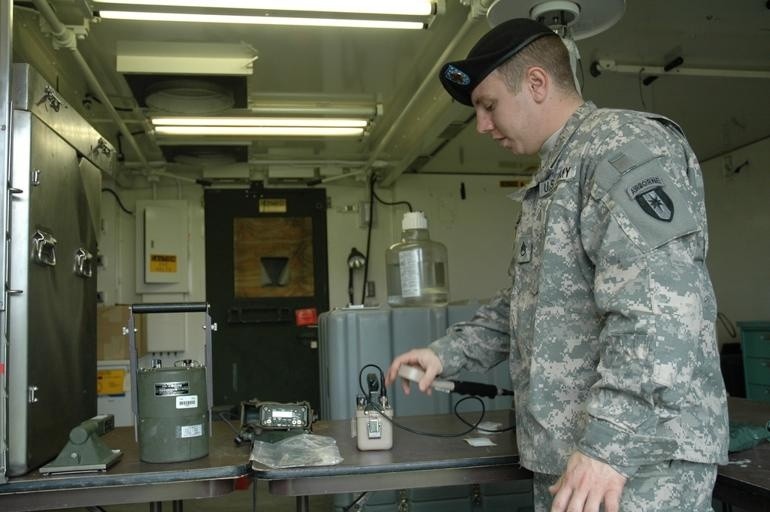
[384,16,732,511]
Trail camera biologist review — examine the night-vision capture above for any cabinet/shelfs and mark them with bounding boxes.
[736,319,770,398]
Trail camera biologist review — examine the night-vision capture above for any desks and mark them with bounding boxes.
[0,421,252,510]
[254,396,770,512]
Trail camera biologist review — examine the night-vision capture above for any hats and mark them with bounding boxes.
[439,18,559,107]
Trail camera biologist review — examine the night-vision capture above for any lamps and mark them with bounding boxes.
[82,1,446,33]
[145,104,383,142]
[344,246,368,309]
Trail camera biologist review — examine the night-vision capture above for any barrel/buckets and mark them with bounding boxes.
[384,211,450,307]
